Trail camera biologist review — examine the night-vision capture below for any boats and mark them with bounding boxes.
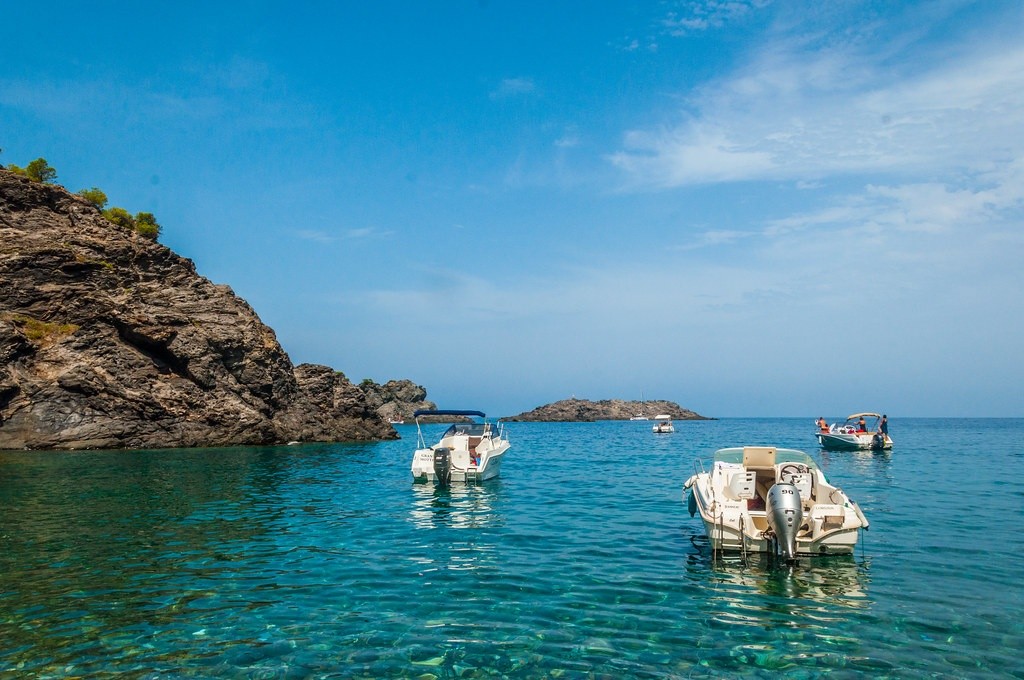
[651,414,674,433]
[690,535,871,663]
[411,409,511,480]
[814,412,894,450]
[683,445,869,564]
[411,480,497,569]
[387,411,404,425]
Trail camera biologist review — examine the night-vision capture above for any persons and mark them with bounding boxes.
[854,417,867,432]
[877,414,888,441]
[817,417,830,433]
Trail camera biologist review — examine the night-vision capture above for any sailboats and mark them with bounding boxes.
[630,391,652,421]
